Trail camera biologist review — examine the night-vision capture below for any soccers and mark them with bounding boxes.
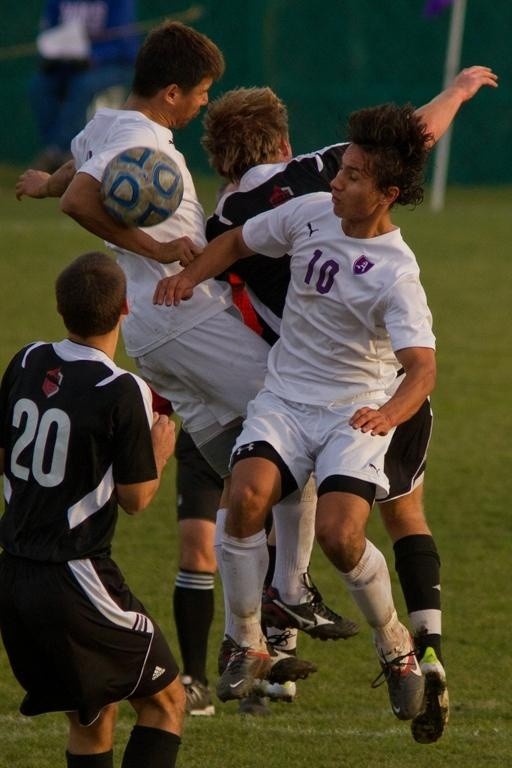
[97,147,183,227]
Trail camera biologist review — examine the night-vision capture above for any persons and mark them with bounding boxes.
[0,250,194,766]
[200,64,504,745]
[27,1,137,176]
[151,101,440,722]
[15,17,361,686]
[143,176,276,714]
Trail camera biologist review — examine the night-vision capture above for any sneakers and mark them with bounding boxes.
[216,578,360,716]
[176,675,214,720]
[376,623,451,742]
